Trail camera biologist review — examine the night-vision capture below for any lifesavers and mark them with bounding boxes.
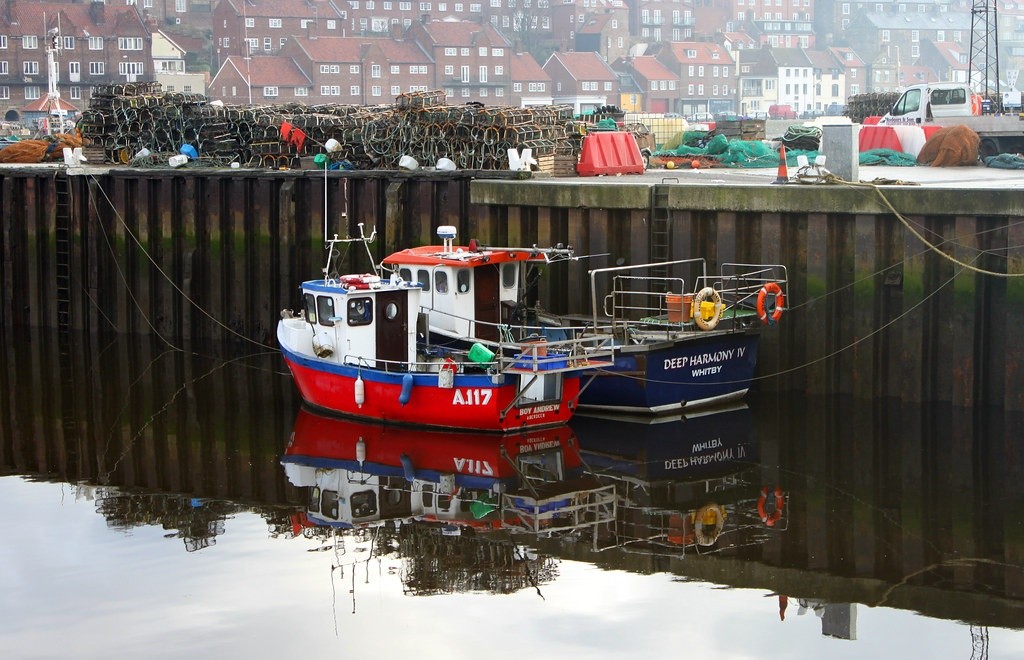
[758,485,785,527]
[341,283,381,289]
[756,283,785,325]
[695,503,725,546]
[339,274,381,283]
[692,286,724,331]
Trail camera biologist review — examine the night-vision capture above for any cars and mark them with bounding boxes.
[799,110,821,119]
[664,112,685,120]
[982,100,992,112]
[687,112,713,121]
[715,112,743,121]
[825,103,851,116]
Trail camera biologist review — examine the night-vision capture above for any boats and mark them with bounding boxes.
[381,226,789,426]
[573,418,791,559]
[279,403,618,632]
[277,162,615,432]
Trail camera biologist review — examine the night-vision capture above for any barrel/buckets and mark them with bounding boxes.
[667,514,694,545]
[665,292,693,323]
[519,333,547,356]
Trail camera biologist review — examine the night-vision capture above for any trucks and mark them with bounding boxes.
[876,82,1024,163]
[768,104,798,120]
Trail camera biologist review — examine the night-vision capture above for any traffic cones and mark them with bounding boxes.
[770,144,801,184]
